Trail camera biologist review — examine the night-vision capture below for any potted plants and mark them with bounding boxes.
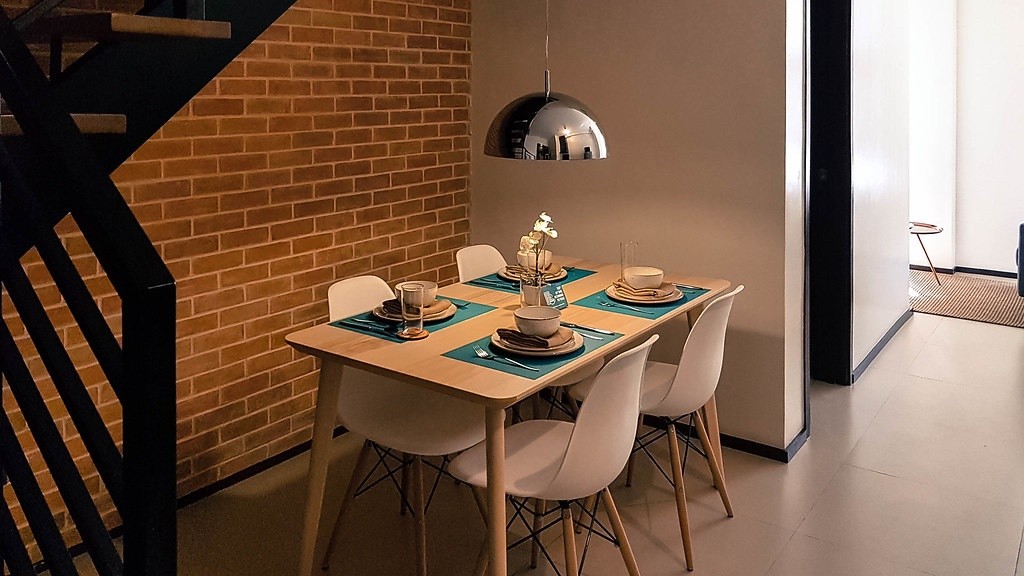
[523,280,551,307]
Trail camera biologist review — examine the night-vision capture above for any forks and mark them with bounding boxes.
[350,318,407,328]
[597,297,654,314]
[473,344,540,372]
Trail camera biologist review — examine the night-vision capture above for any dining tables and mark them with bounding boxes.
[282,254,731,576]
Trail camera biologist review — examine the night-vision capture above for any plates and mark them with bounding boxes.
[605,285,684,303]
[507,270,562,278]
[491,326,584,358]
[614,289,674,301]
[500,337,574,351]
[498,267,567,281]
[382,304,452,319]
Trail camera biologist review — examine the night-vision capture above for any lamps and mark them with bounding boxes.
[483,0,611,162]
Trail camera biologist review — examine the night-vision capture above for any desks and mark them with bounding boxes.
[908,221,943,287]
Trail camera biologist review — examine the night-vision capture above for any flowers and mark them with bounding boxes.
[514,211,558,287]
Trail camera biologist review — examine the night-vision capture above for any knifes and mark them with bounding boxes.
[560,321,615,335]
[340,321,395,338]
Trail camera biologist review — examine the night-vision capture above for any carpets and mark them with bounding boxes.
[909,268,1024,328]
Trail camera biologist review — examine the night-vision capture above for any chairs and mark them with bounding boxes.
[323,276,487,576]
[455,244,582,426]
[446,333,662,576]
[563,284,746,572]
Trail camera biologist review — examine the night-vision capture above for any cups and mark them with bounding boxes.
[401,283,424,335]
[620,241,638,281]
[520,272,541,308]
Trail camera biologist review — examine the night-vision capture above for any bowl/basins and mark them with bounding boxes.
[624,267,663,290]
[517,250,552,271]
[394,281,438,307]
[514,305,562,338]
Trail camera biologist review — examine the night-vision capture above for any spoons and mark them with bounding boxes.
[470,278,530,290]
[376,299,457,322]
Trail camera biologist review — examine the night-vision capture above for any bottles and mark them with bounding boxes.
[583,146,592,159]
